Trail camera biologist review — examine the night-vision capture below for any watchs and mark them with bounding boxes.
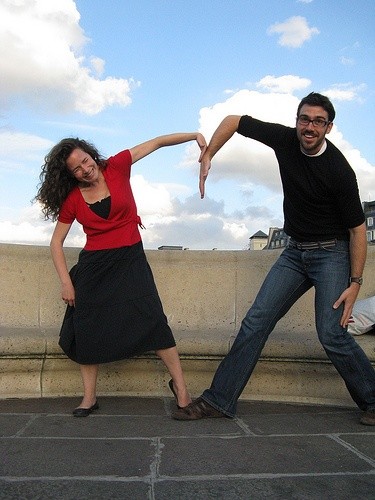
[351,277,363,285]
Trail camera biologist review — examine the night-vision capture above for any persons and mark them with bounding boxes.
[172,93,375,426]
[37,132,208,418]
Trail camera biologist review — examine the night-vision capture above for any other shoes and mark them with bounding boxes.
[72,399,99,417]
[359,411,375,426]
[168,378,193,410]
[170,397,228,420]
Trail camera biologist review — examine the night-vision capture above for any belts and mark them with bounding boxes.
[289,238,338,250]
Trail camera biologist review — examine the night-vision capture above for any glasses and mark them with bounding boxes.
[296,115,331,128]
